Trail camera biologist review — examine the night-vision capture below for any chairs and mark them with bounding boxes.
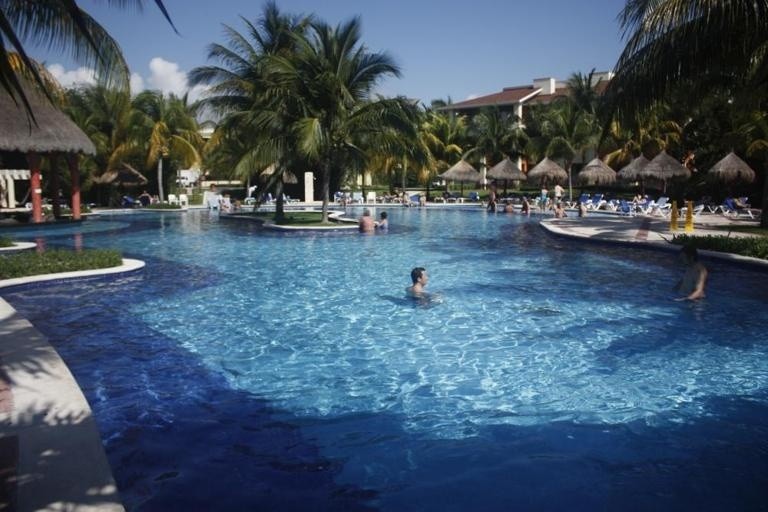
[433,194,753,220]
[167,194,189,208]
[123,195,142,209]
[267,192,300,204]
[335,192,423,203]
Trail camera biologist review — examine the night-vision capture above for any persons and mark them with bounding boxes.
[207,184,241,222]
[405,267,431,295]
[670,244,707,301]
[732,197,763,215]
[138,190,152,206]
[359,210,388,233]
[485,180,651,218]
[340,189,426,207]
